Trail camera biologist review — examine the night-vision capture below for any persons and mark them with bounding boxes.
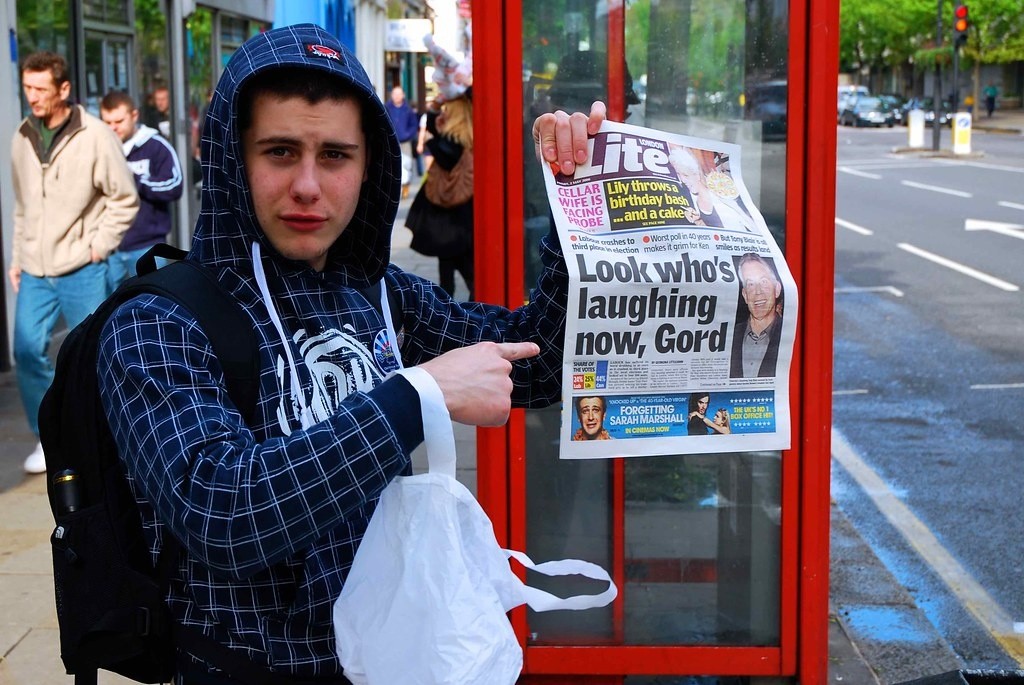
[984,80,998,117]
[572,396,617,441]
[99,91,184,293]
[730,253,784,379]
[687,393,710,435]
[404,72,475,302]
[668,149,758,234]
[688,408,731,435]
[7,53,140,474]
[95,23,608,685]
[526,52,640,642]
[146,87,174,143]
[381,86,417,199]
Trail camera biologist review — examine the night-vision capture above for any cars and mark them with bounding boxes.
[630,75,699,110]
[745,78,787,141]
[836,82,954,128]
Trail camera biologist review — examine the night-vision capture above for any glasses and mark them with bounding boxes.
[624,109,632,120]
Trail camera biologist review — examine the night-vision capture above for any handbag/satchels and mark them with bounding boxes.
[334,367,617,685]
[426,148,475,209]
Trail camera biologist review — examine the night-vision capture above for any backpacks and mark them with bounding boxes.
[38,242,261,684]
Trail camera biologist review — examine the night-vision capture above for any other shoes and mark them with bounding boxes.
[22,440,47,473]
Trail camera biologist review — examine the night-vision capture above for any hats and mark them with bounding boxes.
[545,50,641,106]
[424,34,471,101]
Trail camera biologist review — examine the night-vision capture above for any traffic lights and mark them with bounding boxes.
[952,5,968,46]
